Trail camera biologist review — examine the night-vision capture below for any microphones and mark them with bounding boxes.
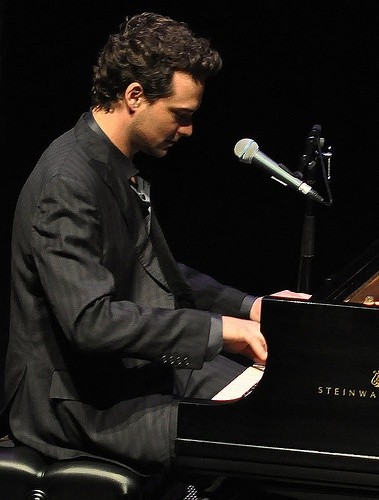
[234,138,326,204]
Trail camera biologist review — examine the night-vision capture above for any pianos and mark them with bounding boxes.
[171,238,379,500]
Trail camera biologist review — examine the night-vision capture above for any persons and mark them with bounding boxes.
[0,10,312,477]
